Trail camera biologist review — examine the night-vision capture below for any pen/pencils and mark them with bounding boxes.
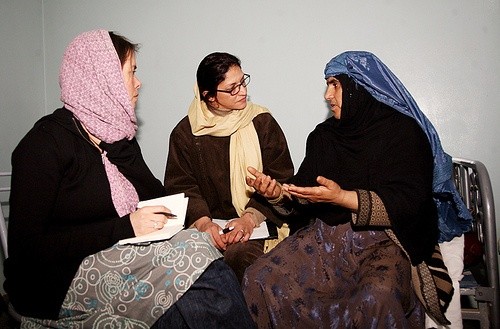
[135,207,177,218]
[219,227,234,235]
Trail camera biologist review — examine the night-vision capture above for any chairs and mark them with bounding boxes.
[0,172,12,258]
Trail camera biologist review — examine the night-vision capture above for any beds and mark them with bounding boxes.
[452,156,500,329]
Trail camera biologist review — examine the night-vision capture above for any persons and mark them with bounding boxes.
[241,50,474,329]
[164,51,294,283]
[3,29,252,329]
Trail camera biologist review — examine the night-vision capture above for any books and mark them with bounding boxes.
[118,193,189,245]
[212,218,278,240]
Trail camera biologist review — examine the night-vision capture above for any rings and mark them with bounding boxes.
[153,222,158,228]
[240,229,244,234]
